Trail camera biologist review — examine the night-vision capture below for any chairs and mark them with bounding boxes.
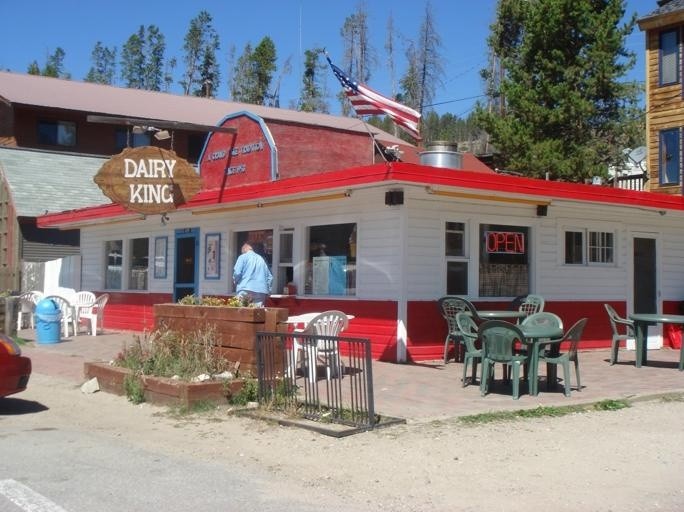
[17,291,109,337]
[293,310,349,384]
[437,294,645,400]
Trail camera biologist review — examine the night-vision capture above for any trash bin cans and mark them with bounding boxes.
[592,175,603,186]
[34,299,62,344]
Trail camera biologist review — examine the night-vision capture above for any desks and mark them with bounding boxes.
[282,313,355,373]
[629,314,684,371]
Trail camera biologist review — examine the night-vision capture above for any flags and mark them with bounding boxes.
[332,62,422,143]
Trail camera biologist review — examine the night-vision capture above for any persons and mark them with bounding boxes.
[231,242,273,309]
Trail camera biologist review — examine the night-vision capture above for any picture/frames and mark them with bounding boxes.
[205,234,220,279]
[154,237,167,278]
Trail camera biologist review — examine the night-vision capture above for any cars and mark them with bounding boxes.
[1,336,31,407]
